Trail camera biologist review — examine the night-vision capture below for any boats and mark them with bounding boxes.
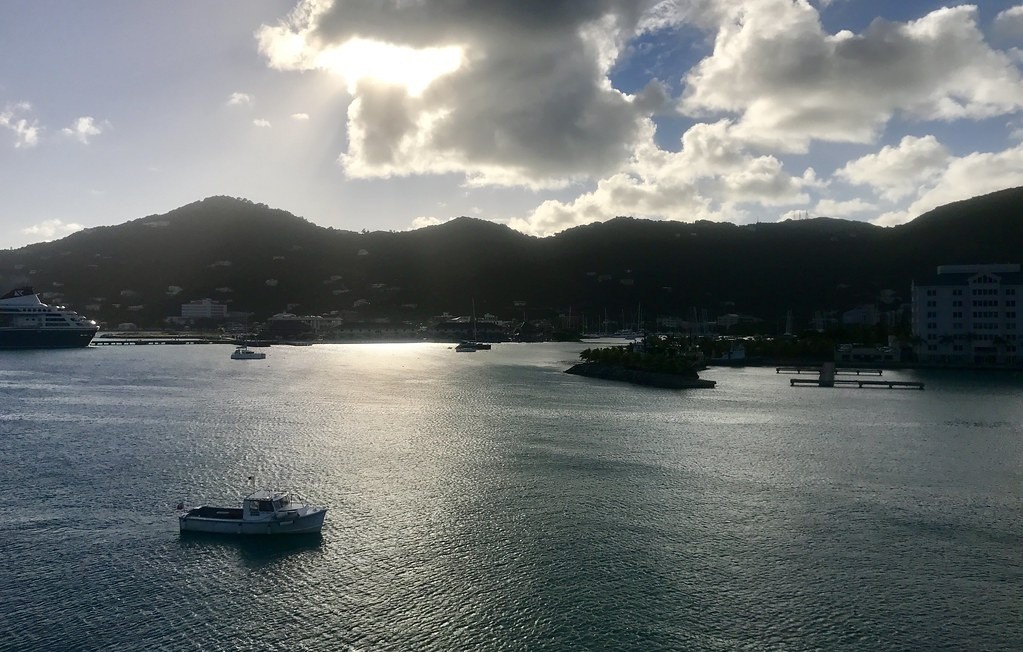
[0,286,100,348]
[178,474,329,535]
[230,312,266,360]
[454,294,491,352]
